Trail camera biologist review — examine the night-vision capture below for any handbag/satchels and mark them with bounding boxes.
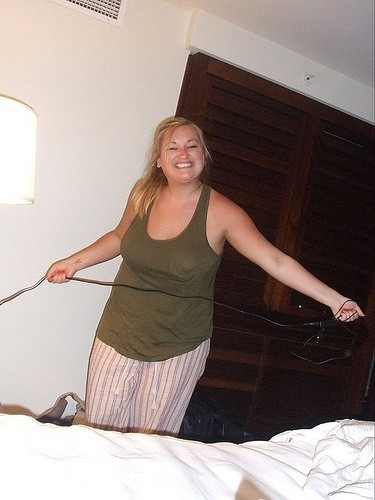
[72,400,87,425]
[182,404,215,439]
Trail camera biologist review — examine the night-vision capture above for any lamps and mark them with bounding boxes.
[0,94,37,206]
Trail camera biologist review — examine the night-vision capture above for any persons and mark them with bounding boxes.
[45,117,365,438]
